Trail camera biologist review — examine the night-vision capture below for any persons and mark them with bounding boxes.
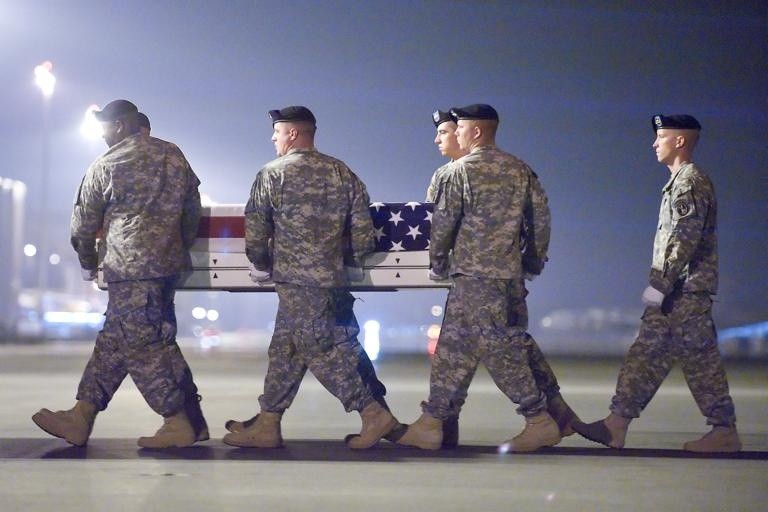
[31,98,202,453]
[221,105,399,451]
[386,102,563,454]
[226,168,404,434]
[40,111,211,444]
[572,111,744,455]
[424,105,582,446]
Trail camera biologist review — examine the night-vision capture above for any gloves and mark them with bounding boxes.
[347,266,364,282]
[429,268,445,280]
[641,285,665,308]
[81,269,97,281]
[524,273,537,282]
[250,263,270,282]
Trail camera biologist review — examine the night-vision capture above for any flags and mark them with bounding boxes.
[185,202,446,253]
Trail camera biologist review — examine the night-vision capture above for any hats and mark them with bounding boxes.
[137,112,150,128]
[432,109,457,127]
[651,113,701,135]
[268,106,316,124]
[95,100,138,121]
[449,104,499,120]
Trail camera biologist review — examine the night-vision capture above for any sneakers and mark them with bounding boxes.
[501,410,562,451]
[32,400,99,446]
[383,407,443,450]
[138,407,196,447]
[548,395,581,437]
[226,413,260,432]
[443,418,459,449]
[345,434,361,443]
[185,394,210,442]
[222,408,284,448]
[571,412,632,450]
[347,399,401,449]
[683,427,742,453]
